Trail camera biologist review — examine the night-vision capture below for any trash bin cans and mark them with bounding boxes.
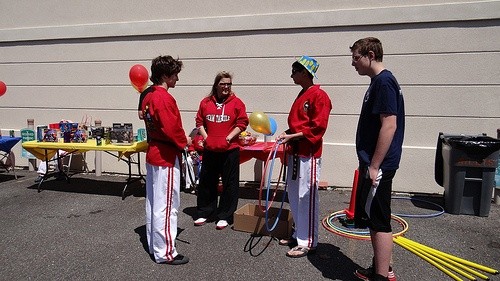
[440,132,499,217]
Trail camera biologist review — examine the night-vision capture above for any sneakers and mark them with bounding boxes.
[353,264,396,281]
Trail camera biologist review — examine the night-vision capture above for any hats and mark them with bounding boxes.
[294,55,321,83]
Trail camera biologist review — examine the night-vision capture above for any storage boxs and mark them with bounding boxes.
[233,203,294,239]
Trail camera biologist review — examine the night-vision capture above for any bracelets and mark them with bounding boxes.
[226,136,230,142]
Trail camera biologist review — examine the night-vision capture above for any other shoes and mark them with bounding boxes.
[216,220,229,230]
[194,218,207,225]
[168,254,189,265]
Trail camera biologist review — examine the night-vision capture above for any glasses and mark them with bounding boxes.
[292,69,298,74]
[353,51,368,62]
[218,82,232,86]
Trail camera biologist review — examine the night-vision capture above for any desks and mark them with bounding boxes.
[0,135,22,180]
[22,138,149,200]
[189,133,293,166]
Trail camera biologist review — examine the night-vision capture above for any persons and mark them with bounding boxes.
[142,55,192,265]
[194,71,249,229]
[138,85,151,119]
[345,37,405,281]
[274,55,332,257]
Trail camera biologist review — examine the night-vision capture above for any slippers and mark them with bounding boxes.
[280,237,297,245]
[286,247,316,258]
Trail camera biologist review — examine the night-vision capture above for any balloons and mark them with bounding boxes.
[249,111,271,136]
[0,81,7,96]
[266,117,277,136]
[129,64,148,93]
